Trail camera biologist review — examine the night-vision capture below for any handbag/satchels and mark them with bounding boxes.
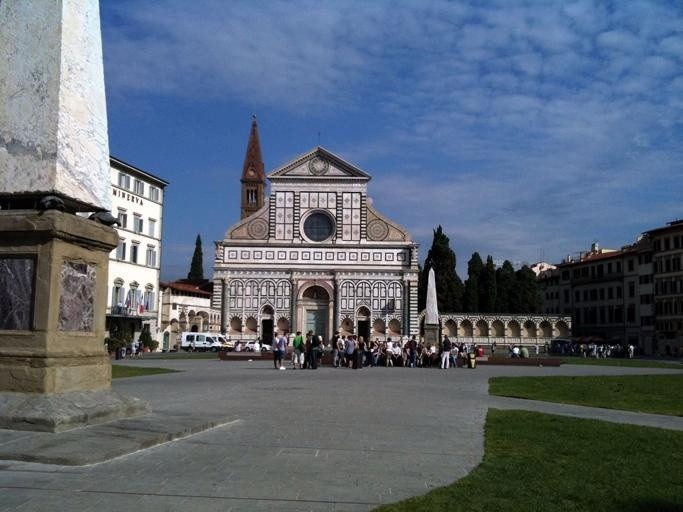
[299,335,307,353]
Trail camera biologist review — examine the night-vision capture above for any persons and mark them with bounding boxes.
[188,339,194,353]
[234,340,242,352]
[129,340,145,359]
[117,301,122,314]
[257,337,263,349]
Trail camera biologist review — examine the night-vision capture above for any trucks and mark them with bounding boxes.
[206,333,242,352]
[181,331,220,352]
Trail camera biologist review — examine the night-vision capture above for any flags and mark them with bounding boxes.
[140,295,144,314]
[127,292,132,315]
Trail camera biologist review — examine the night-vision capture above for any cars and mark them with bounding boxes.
[243,341,270,353]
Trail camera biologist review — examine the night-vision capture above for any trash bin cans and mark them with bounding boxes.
[116,347,122,359]
[467,352,476,369]
